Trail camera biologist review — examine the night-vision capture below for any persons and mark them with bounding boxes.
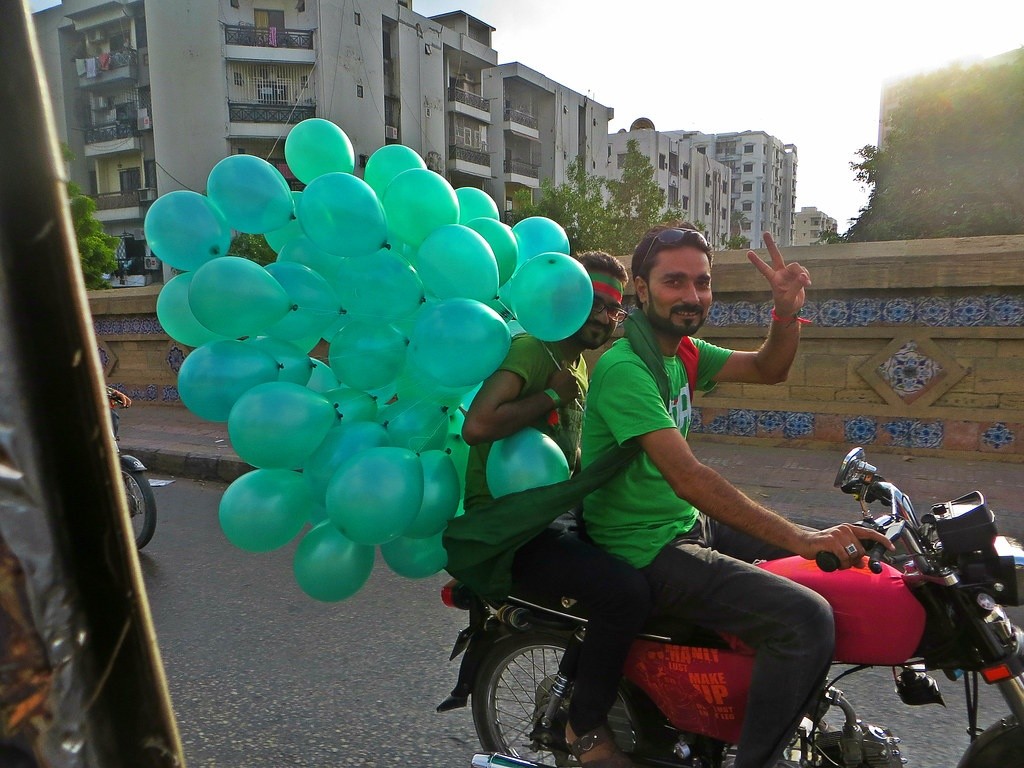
[461,245,653,768]
[105,385,131,409]
[579,217,896,768]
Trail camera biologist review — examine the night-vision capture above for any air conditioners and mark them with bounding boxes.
[386,125,398,140]
[143,256,161,271]
[137,116,152,130]
[88,29,106,43]
[139,190,156,202]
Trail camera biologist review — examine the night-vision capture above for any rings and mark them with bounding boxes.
[845,544,857,554]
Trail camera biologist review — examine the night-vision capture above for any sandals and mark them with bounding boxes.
[565,725,633,767]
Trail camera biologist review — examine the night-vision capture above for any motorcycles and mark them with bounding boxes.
[440,446,1024,768]
[106,388,157,550]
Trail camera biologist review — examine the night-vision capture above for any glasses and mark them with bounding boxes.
[592,299,628,323]
[642,228,709,263]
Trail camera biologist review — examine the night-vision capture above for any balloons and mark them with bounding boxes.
[143,119,596,604]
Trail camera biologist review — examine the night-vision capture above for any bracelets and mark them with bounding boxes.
[772,304,812,325]
[545,387,561,407]
